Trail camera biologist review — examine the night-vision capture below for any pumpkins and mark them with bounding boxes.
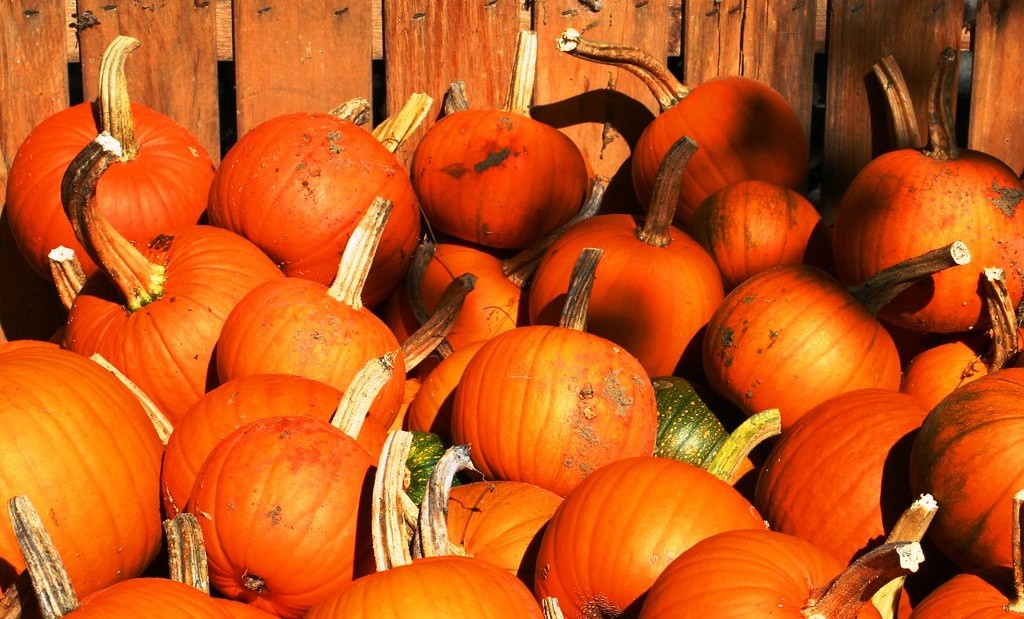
[0,36,1024,619]
